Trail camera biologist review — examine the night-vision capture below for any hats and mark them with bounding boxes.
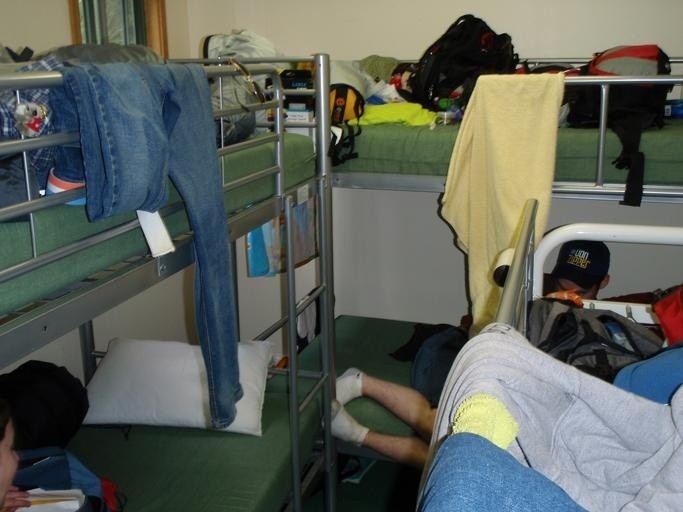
[551,240,611,290]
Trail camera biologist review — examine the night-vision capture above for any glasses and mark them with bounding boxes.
[228,57,266,113]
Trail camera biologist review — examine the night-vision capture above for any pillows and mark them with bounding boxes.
[83,337,271,438]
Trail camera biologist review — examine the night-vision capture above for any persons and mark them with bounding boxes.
[321,367,434,470]
[543,242,610,300]
[0,399,31,512]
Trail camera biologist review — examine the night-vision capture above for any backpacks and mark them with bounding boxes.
[515,45,674,207]
[525,298,664,385]
[11,446,127,512]
[203,29,255,148]
[391,14,520,112]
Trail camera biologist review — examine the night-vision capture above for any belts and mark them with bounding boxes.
[45,167,176,258]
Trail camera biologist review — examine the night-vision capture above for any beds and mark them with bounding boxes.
[418,201,683,512]
[0,53,337,512]
[231,36,683,475]
